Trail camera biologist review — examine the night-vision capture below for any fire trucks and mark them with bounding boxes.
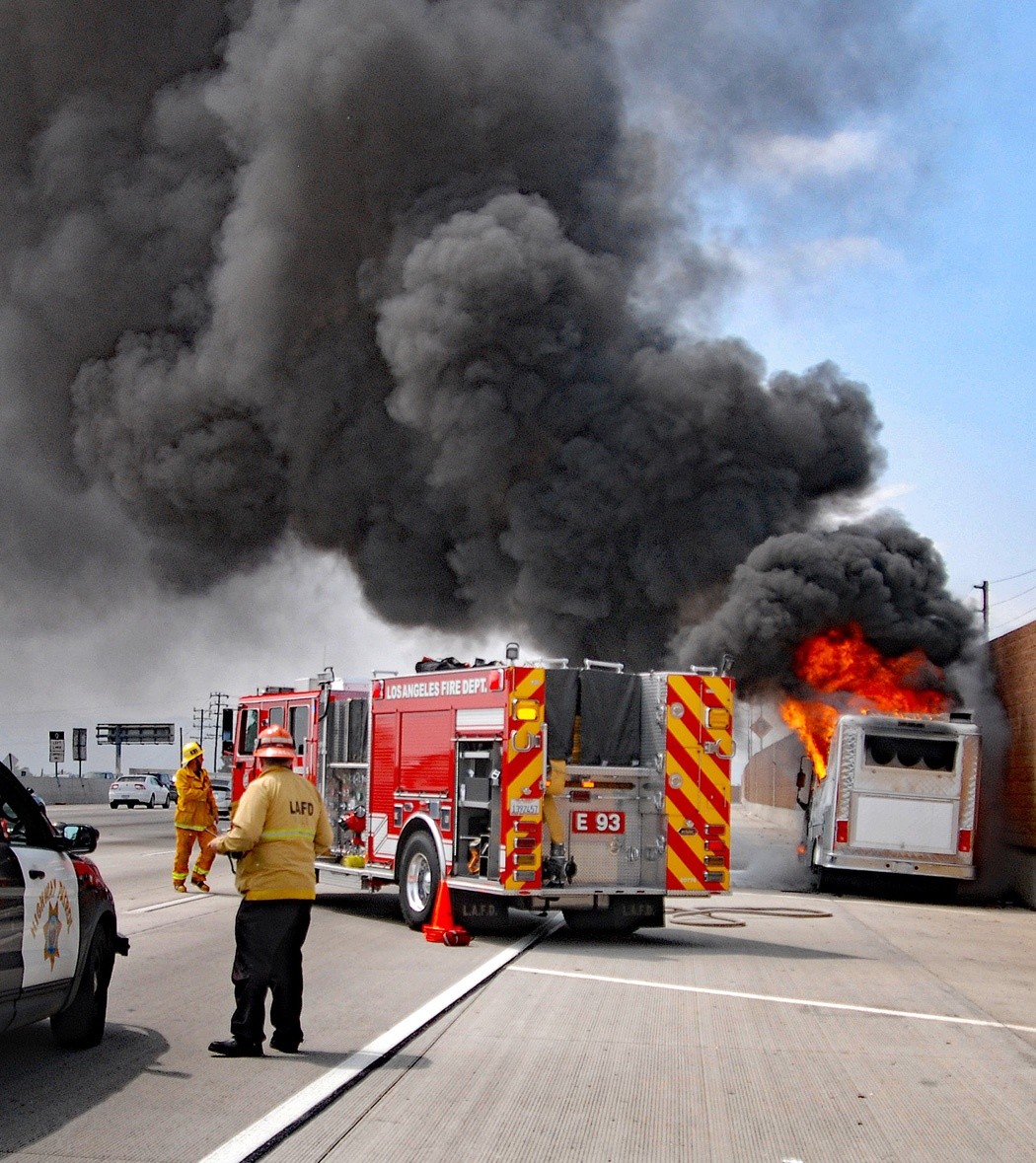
[791,705,985,906]
[221,640,738,940]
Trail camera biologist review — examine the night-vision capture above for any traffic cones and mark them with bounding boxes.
[422,880,467,945]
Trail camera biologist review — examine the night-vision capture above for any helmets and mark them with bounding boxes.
[181,742,204,765]
[253,725,299,759]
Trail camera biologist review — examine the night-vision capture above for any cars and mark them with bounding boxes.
[0,761,131,1050]
[211,785,232,815]
[3,790,47,833]
[83,772,114,779]
[108,774,171,809]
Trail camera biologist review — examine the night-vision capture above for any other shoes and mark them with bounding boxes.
[174,885,187,893]
[190,879,210,892]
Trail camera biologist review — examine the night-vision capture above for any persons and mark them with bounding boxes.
[172,743,220,896]
[205,725,333,1057]
[246,710,257,751]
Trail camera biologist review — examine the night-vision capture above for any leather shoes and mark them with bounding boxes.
[270,1039,299,1053]
[208,1036,264,1057]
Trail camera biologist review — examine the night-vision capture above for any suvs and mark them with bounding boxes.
[142,771,179,803]
